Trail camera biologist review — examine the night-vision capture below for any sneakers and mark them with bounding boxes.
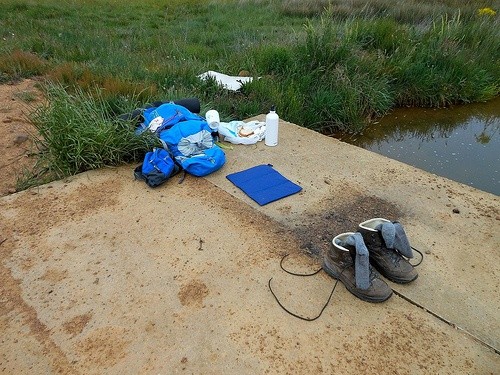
[321,232,392,302]
[357,216,419,283]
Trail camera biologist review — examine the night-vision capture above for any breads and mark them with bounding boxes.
[238,127,254,138]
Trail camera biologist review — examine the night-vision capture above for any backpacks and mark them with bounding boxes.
[160,120,226,183]
